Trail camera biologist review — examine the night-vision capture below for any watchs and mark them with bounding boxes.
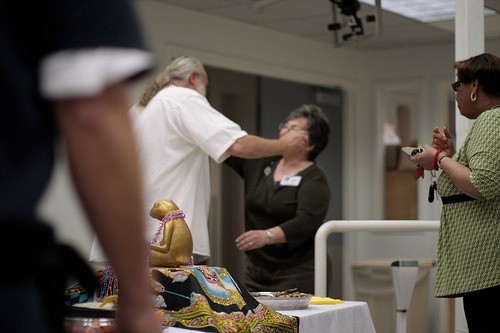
[266,229,274,245]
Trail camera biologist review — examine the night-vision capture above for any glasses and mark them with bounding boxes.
[278,121,307,133]
[451,79,464,91]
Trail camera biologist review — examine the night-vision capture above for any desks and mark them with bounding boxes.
[62,289,376,333]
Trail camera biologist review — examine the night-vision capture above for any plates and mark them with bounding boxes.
[249,292,311,310]
[402,147,424,155]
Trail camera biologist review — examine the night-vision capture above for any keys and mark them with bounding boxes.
[428,181,438,203]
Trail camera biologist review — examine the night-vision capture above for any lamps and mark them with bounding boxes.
[383,123,403,172]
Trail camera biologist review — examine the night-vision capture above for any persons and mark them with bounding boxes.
[223,104,333,295]
[0,0,166,333]
[89,56,308,265]
[410,53,500,333]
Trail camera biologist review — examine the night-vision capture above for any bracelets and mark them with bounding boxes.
[438,156,446,170]
[432,150,440,171]
